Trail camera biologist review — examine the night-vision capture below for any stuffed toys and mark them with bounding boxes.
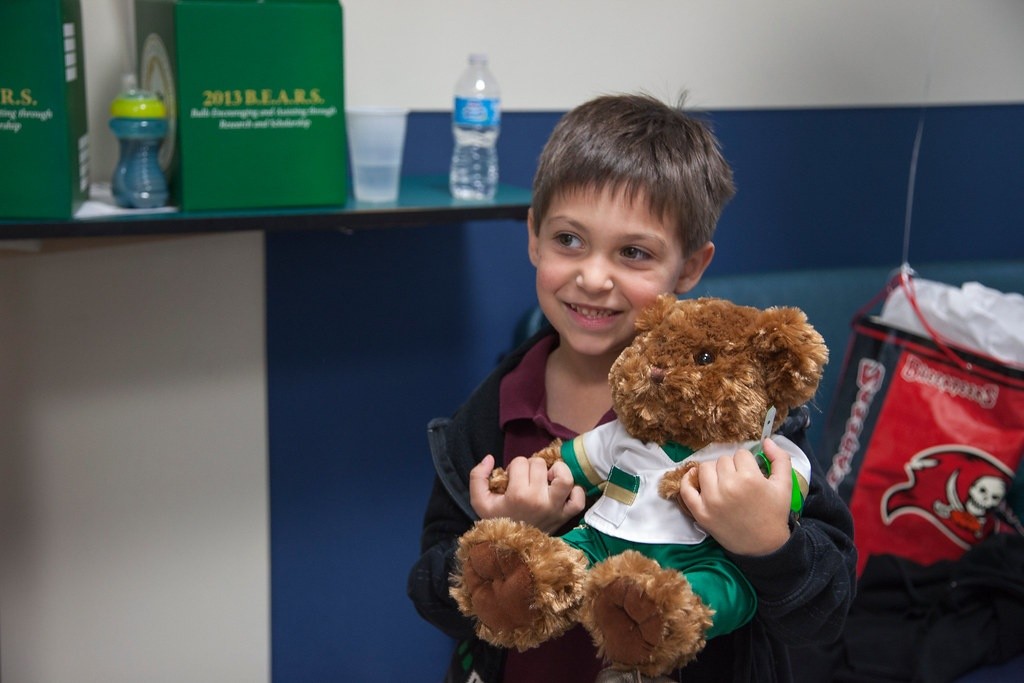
[449,293,828,677]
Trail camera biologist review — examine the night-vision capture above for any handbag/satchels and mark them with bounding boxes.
[828,271,1024,583]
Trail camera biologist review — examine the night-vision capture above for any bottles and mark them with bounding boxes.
[448,53,502,200]
[108,74,170,209]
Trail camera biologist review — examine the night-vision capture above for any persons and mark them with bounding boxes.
[406,96,856,683]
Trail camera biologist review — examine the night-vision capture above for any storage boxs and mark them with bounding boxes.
[0,0,89,218]
[132,0,346,211]
